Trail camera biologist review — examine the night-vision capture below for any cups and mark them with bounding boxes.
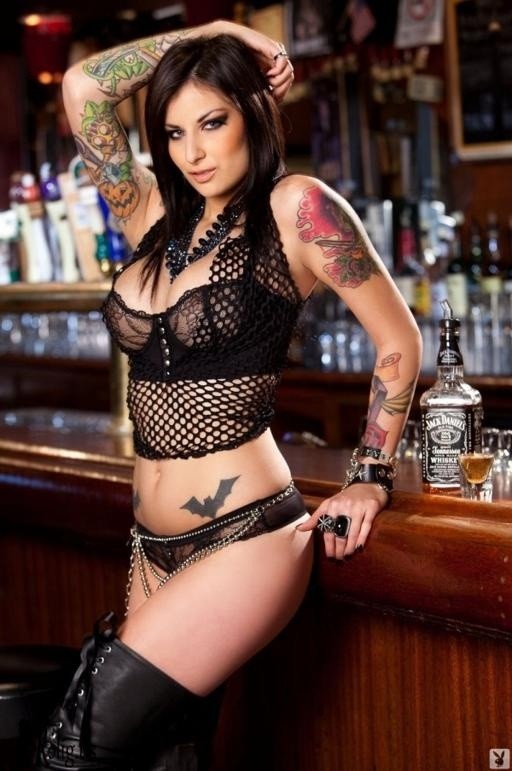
[455,454,495,502]
[302,318,512,472]
[0,310,113,362]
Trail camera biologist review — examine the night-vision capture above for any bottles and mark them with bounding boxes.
[386,201,512,324]
[419,299,485,498]
[0,152,132,286]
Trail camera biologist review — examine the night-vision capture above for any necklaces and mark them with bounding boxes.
[164,196,246,286]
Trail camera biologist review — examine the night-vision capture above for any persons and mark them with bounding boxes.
[35,18,423,770]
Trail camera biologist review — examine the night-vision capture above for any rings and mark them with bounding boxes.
[317,513,334,533]
[332,515,352,538]
[282,54,289,58]
[272,52,284,60]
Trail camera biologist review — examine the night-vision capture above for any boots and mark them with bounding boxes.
[41,634,223,771]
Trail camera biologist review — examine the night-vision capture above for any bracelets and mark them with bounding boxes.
[342,445,399,495]
